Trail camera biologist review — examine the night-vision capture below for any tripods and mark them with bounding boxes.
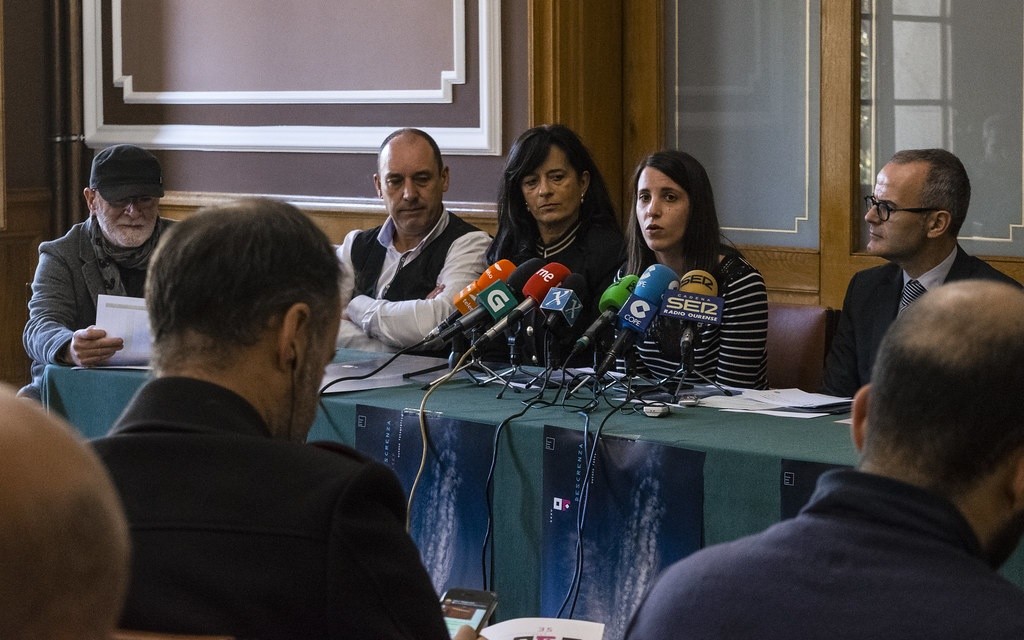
[403,321,733,405]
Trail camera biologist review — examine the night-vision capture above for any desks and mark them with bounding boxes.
[36,346,866,640]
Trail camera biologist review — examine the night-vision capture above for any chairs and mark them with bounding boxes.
[764,305,841,392]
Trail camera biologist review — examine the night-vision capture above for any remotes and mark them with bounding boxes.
[678,394,699,405]
[644,405,674,417]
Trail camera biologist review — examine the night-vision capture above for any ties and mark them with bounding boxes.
[898,280,926,315]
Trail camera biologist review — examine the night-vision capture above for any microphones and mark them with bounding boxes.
[424,257,725,379]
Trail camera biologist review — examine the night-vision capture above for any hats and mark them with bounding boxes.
[90,145,164,202]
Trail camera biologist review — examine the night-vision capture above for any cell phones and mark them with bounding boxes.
[439,588,498,640]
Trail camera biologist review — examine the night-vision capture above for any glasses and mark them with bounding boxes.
[864,195,936,222]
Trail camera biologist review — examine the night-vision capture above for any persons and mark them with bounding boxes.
[331,129,494,356]
[622,278,1024,640]
[15,144,175,403]
[822,149,1024,399]
[475,124,628,372]
[614,150,768,391]
[87,198,489,640]
[0,381,130,640]
